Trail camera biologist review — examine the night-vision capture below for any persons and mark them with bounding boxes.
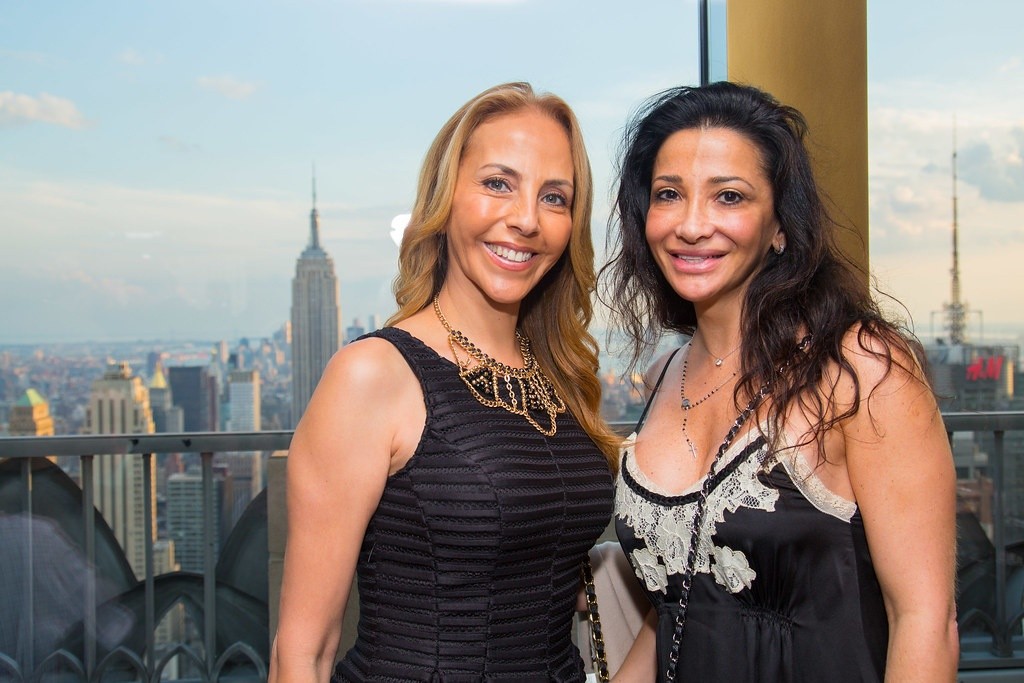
[610,80,961,683]
[267,81,619,683]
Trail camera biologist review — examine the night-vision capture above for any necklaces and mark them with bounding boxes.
[434,292,566,436]
[681,328,742,457]
